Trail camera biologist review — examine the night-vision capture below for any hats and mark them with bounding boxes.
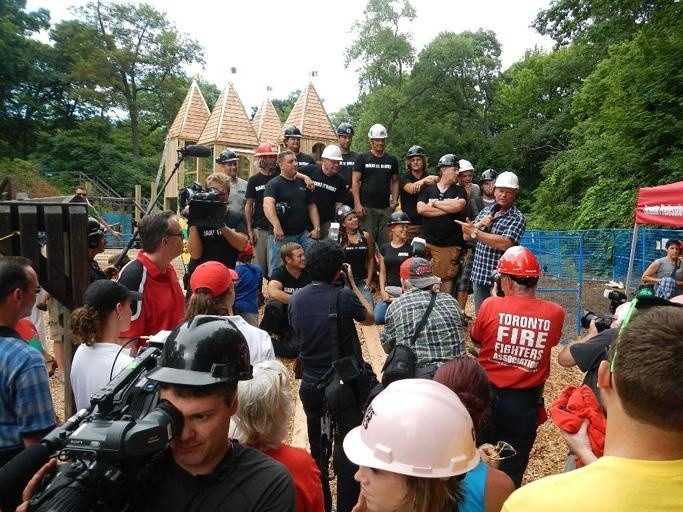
[398,256,442,290]
[188,259,240,297]
[238,244,255,259]
[82,280,145,320]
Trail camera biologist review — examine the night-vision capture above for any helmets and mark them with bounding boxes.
[284,125,303,138]
[215,149,240,165]
[145,315,254,390]
[480,168,500,182]
[274,202,289,219]
[496,246,542,279]
[336,205,355,222]
[367,123,388,139]
[436,153,460,169]
[387,211,411,226]
[335,123,355,136]
[342,377,481,480]
[253,142,279,157]
[88,219,106,251]
[457,158,475,173]
[406,144,426,157]
[321,144,343,161]
[493,171,520,189]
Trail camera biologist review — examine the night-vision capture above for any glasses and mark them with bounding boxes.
[29,286,42,294]
[480,441,517,461]
[608,293,682,389]
[166,232,185,239]
[76,193,87,196]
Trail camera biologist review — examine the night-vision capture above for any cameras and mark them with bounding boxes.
[0,329,184,512]
[490,270,504,295]
[178,182,228,230]
[606,290,626,313]
[581,308,616,331]
[333,267,346,288]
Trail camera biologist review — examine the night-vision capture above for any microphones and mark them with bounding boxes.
[481,204,501,231]
[176,144,210,158]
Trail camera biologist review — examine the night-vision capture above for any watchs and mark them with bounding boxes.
[469,229,478,241]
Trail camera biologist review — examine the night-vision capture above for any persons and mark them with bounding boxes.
[452,171,526,318]
[76,186,120,240]
[283,127,314,163]
[15,316,295,510]
[259,242,309,355]
[417,154,468,295]
[86,217,108,283]
[233,242,263,326]
[262,150,320,276]
[337,205,378,308]
[469,245,565,488]
[336,123,358,186]
[642,240,683,299]
[287,240,375,510]
[183,173,249,302]
[296,145,355,240]
[48,293,66,383]
[244,142,280,279]
[218,149,246,213]
[380,259,468,379]
[71,280,143,413]
[433,356,515,512]
[29,227,57,378]
[228,359,324,512]
[500,305,682,510]
[343,379,479,512]
[399,143,439,224]
[118,212,186,357]
[187,261,276,366]
[557,295,669,423]
[351,124,399,243]
[456,168,497,327]
[375,211,414,323]
[459,159,481,199]
[1,255,57,468]
[558,415,598,468]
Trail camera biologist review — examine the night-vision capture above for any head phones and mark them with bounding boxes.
[88,225,103,249]
